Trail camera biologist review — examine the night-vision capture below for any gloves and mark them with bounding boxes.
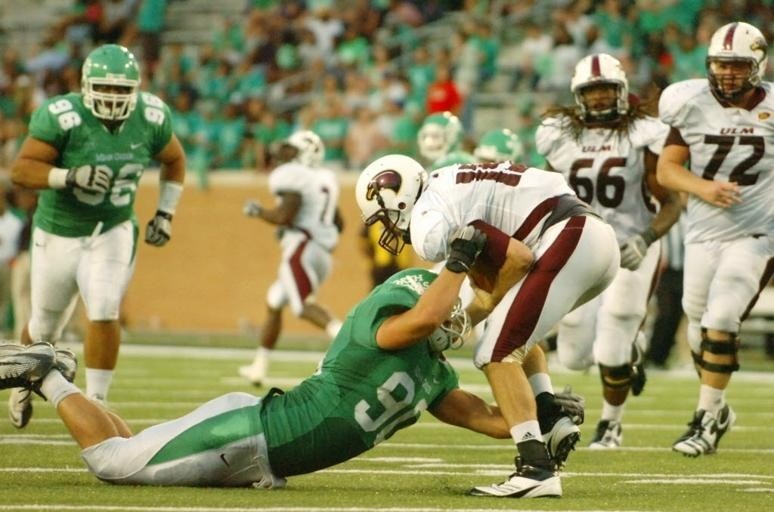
[45,166,112,195]
[555,392,584,425]
[620,228,660,272]
[144,181,184,247]
[445,226,486,274]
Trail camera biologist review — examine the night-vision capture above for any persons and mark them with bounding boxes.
[415,110,478,172]
[425,0,522,115]
[235,129,344,386]
[141,1,773,169]
[0,224,586,490]
[522,0,580,167]
[7,43,188,429]
[580,0,774,95]
[0,0,34,330]
[533,51,683,449]
[655,21,774,458]
[354,153,622,499]
[35,0,140,111]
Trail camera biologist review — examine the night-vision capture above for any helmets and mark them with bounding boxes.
[355,154,429,253]
[283,131,325,164]
[706,22,767,98]
[417,112,464,159]
[385,268,470,354]
[571,53,629,124]
[81,43,140,123]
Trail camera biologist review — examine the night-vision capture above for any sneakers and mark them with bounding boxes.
[673,410,718,458]
[1,345,77,383]
[8,387,47,429]
[471,457,563,500]
[545,413,580,472]
[587,419,624,449]
[239,365,263,386]
[714,401,737,442]
[631,343,646,396]
[1,342,56,393]
